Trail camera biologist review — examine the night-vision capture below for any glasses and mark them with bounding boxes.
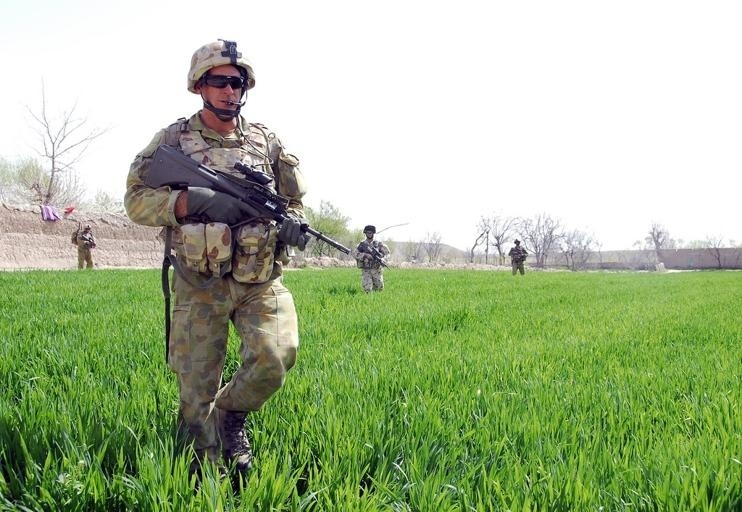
[204,74,245,90]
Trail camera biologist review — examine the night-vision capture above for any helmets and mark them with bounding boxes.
[186,38,256,94]
[363,225,376,234]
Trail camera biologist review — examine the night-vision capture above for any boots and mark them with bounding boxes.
[216,408,254,475]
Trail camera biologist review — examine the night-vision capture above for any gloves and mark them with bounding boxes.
[277,216,308,252]
[187,186,259,224]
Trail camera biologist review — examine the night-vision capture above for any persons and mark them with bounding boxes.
[125,39,309,493]
[76,223,96,271]
[353,225,390,294]
[509,239,528,276]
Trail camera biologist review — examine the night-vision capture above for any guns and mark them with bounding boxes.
[511,248,523,256]
[144,144,351,255]
[357,241,390,270]
[81,234,97,248]
